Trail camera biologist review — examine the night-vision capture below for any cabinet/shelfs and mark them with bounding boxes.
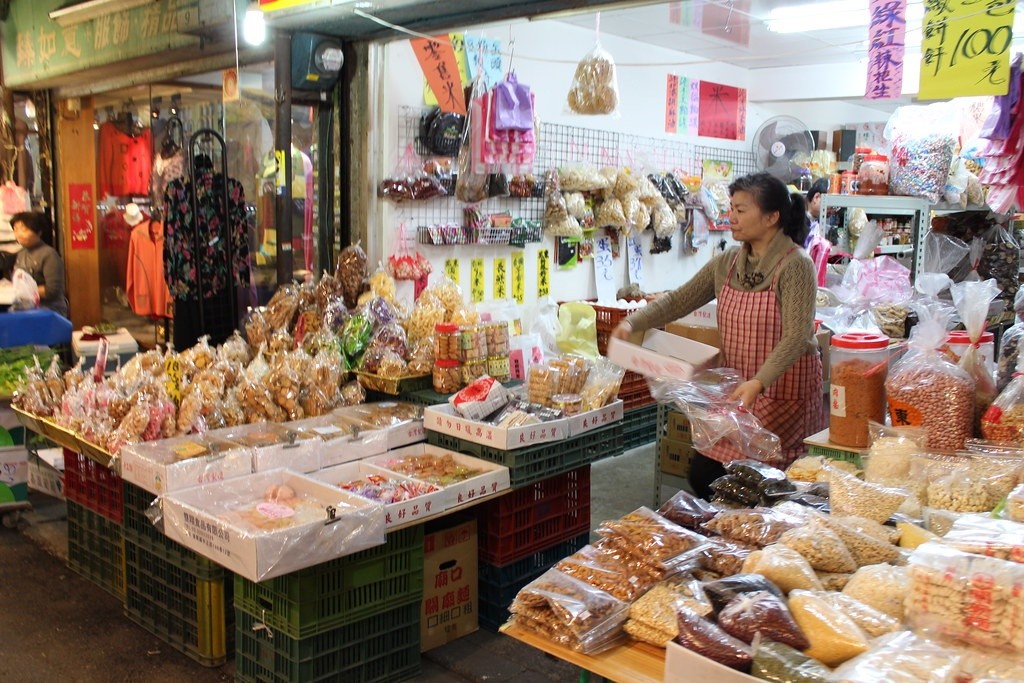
[820,193,1013,284]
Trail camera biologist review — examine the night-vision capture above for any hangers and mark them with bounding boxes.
[113,107,144,136]
[149,203,163,224]
[188,146,214,175]
[160,126,179,159]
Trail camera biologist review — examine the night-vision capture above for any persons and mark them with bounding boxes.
[102,201,153,276]
[10,212,69,320]
[100,111,153,197]
[611,172,822,503]
[797,176,828,249]
[162,157,254,352]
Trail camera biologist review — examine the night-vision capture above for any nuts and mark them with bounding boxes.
[884,368,1024,451]
[507,512,699,650]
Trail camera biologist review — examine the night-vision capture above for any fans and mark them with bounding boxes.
[749,114,815,182]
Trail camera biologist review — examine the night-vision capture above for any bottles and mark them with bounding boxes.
[432,322,511,394]
[826,170,841,194]
[841,170,857,195]
[946,330,994,379]
[876,217,911,245]
[858,155,890,196]
[853,148,872,174]
[830,332,888,447]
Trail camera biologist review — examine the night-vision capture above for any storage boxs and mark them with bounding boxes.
[9,305,829,683]
[664,641,769,682]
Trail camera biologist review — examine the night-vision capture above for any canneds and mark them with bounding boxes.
[829,147,871,195]
[457,321,512,388]
[874,217,911,245]
[432,323,462,394]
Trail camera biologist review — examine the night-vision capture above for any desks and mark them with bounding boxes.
[499,624,667,683]
[0,310,73,366]
[801,419,891,455]
